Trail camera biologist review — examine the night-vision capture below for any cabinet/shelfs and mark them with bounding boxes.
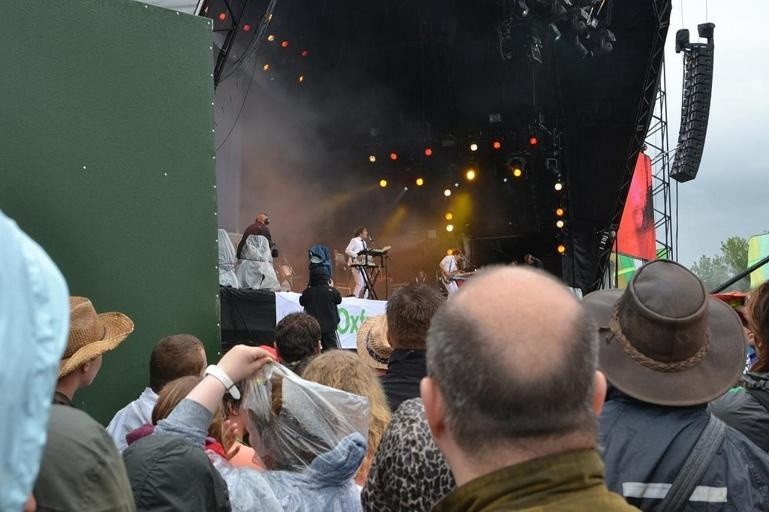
[351,250,387,300]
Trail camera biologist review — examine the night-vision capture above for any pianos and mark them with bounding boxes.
[350,264,388,269]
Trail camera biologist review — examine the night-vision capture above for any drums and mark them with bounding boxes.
[278,264,293,278]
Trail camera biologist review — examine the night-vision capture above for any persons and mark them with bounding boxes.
[1,208,767,512]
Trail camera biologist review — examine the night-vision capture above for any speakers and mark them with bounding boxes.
[669,47,714,183]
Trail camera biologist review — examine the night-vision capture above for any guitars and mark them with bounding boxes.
[443,267,478,284]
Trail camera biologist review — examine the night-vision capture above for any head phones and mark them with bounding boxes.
[256,213,270,224]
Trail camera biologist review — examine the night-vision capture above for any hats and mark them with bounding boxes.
[581,259,747,407]
[357,315,393,370]
[57,296,134,381]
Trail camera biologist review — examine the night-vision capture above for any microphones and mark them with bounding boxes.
[368,235,375,245]
[531,256,541,262]
